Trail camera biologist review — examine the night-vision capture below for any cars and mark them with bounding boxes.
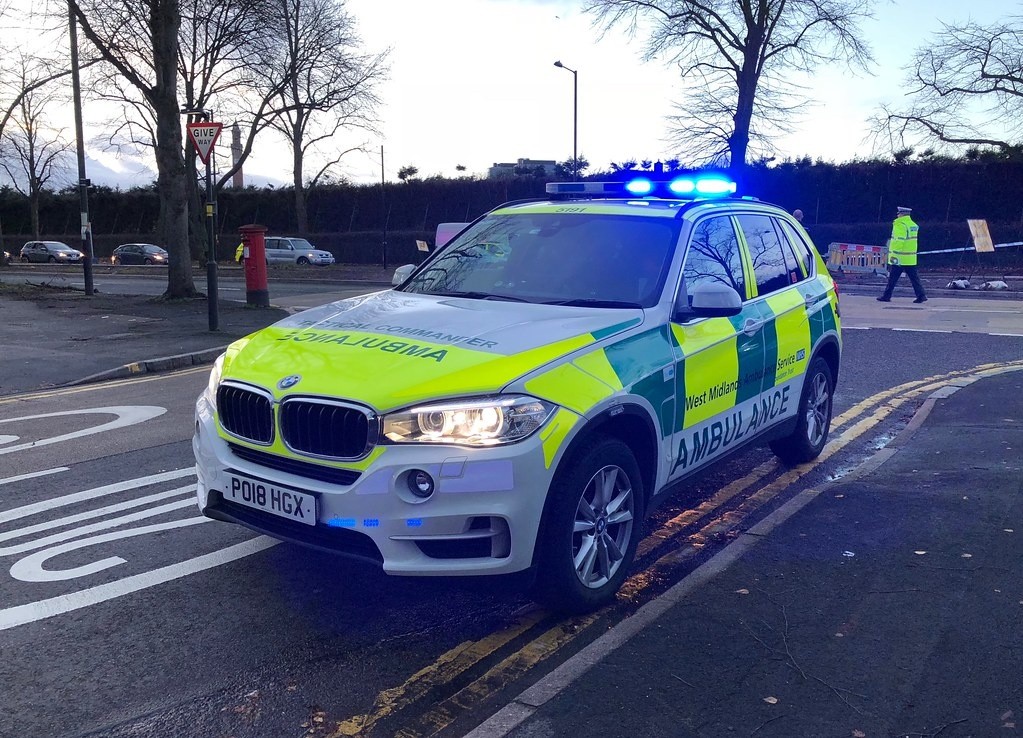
[235,243,244,265]
[112,243,169,265]
[4,251,13,262]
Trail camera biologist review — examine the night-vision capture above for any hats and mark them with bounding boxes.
[896,206,912,213]
[793,210,803,220]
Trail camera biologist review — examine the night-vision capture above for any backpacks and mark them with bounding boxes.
[804,224,810,235]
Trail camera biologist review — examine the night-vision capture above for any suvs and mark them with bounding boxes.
[20,240,84,263]
[264,236,335,267]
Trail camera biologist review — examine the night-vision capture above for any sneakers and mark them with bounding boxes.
[913,296,928,303]
[876,296,890,302]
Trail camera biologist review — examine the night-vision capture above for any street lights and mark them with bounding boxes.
[554,60,577,181]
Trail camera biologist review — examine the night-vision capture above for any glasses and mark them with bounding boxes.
[897,211,900,215]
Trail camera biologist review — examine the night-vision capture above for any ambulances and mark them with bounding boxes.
[192,181,843,610]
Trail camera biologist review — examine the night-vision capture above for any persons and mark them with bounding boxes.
[876,207,929,304]
[793,210,812,237]
